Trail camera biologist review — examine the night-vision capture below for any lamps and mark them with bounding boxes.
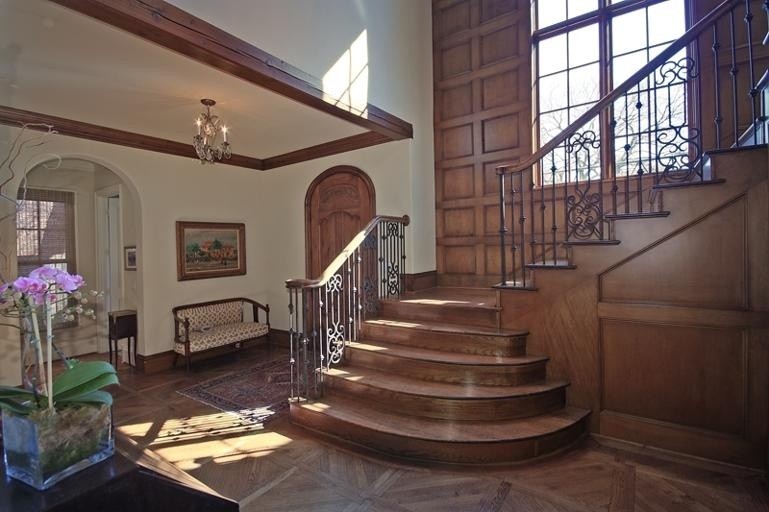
[193,98,232,163]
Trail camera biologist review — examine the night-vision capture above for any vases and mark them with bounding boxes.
[2,403,116,492]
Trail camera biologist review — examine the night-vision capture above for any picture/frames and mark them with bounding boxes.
[176,220,247,282]
[122,246,138,272]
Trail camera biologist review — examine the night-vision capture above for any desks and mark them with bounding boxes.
[108,309,137,371]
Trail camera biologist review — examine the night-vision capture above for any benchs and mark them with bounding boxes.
[168,297,272,374]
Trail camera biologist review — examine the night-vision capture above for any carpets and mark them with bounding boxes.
[176,354,319,425]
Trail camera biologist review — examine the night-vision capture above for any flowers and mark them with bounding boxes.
[0,265,104,419]
